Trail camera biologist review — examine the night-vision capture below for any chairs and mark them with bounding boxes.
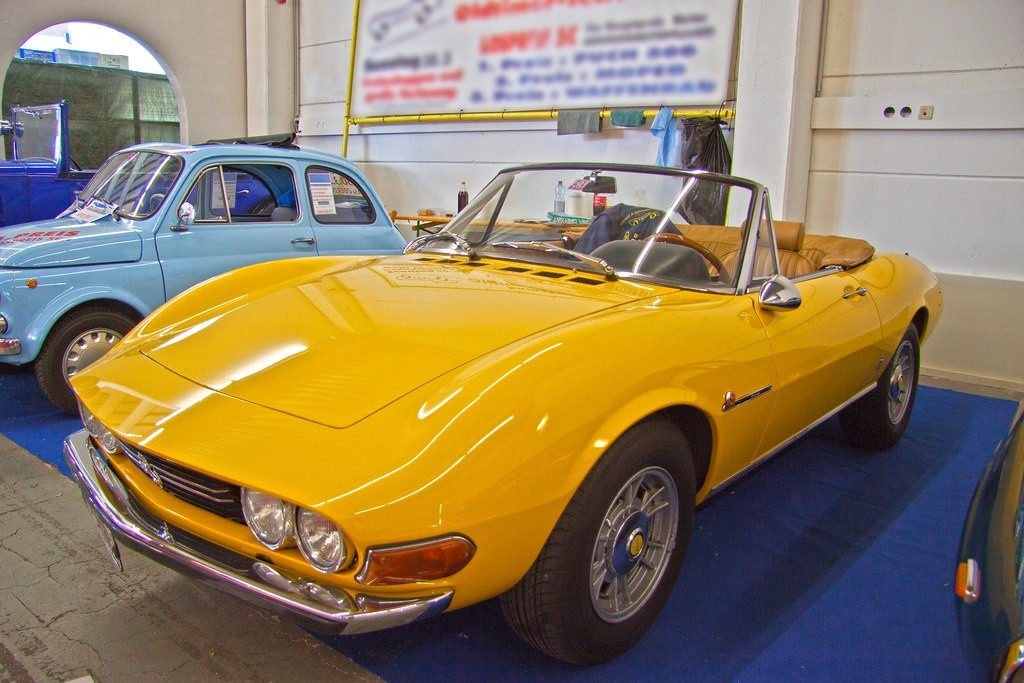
[567,202,683,260]
[709,219,816,283]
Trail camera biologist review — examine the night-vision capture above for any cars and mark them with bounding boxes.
[952,389,1023,683]
[1,140,410,419]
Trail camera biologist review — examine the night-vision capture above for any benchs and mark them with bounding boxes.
[673,224,875,270]
[270,207,369,222]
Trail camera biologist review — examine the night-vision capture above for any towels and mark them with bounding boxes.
[556,110,604,136]
[611,107,647,128]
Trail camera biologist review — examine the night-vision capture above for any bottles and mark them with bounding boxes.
[554,181,565,214]
[593,193,607,219]
[458,181,468,214]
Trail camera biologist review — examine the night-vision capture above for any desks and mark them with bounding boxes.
[396,215,588,238]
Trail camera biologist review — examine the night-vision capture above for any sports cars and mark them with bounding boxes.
[63,154,946,668]
